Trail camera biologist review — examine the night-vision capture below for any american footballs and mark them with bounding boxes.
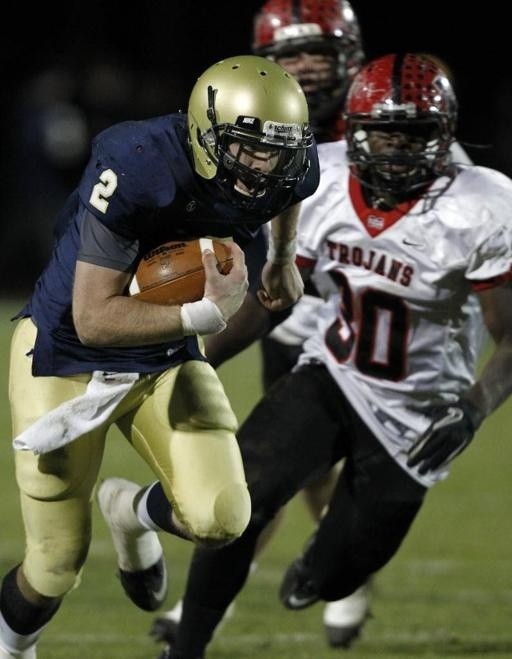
[127,240,232,307]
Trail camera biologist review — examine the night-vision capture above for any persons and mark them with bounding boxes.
[3,56,321,656]
[166,51,512,659]
[0,48,512,396]
[251,0,476,652]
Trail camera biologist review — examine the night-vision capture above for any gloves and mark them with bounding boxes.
[405,398,486,476]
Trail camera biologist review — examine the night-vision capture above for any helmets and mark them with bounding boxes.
[343,53,460,193]
[187,55,313,211]
[252,0,363,118]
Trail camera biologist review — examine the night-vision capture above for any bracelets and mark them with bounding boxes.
[178,296,227,338]
[267,230,297,266]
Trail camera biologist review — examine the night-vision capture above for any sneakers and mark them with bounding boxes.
[282,554,373,647]
[95,476,166,613]
[150,599,185,646]
